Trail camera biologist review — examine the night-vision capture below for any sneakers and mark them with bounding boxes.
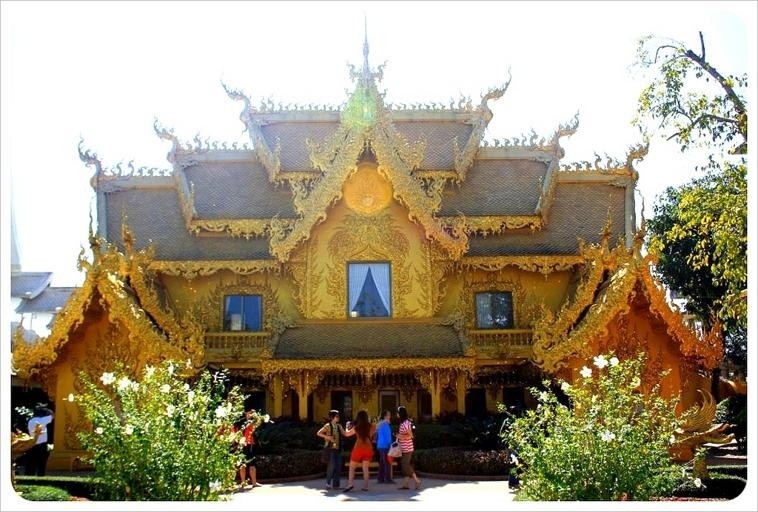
[417,479,422,490]
[253,483,261,487]
[377,479,396,484]
[361,488,368,491]
[325,484,354,493]
[398,487,410,490]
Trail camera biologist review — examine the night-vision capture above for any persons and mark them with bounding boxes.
[392,406,421,489]
[343,410,375,492]
[374,409,395,484]
[237,408,261,487]
[499,406,524,489]
[24,403,53,477]
[211,415,241,454]
[316,410,345,490]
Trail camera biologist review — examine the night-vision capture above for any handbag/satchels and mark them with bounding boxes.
[387,441,403,457]
[321,448,330,463]
